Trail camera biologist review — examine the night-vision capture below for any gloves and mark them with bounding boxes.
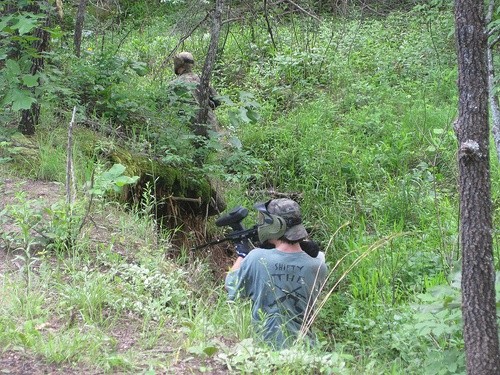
[234,240,249,258]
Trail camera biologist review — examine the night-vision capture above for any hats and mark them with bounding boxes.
[266,198,309,240]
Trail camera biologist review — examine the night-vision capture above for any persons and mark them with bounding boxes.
[225,198,326,348]
[169,52,217,135]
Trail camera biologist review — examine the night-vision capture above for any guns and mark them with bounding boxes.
[189,207,320,260]
